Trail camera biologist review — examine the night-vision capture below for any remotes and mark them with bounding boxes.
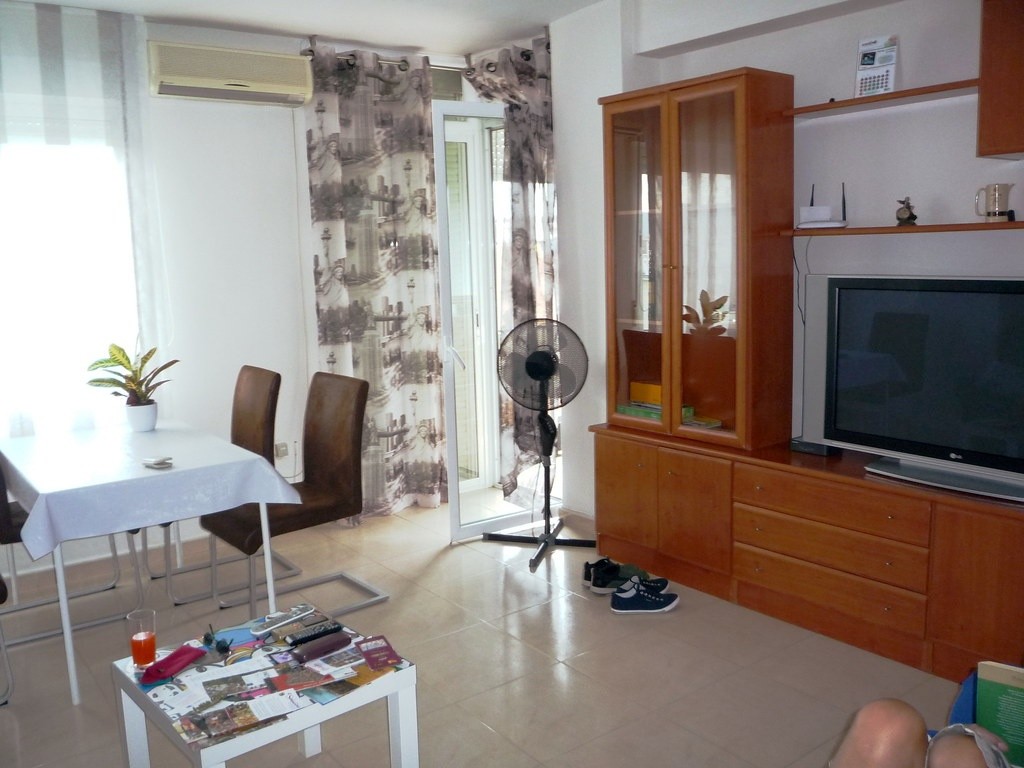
[249,603,341,646]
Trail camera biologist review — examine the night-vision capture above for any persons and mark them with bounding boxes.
[828,697,1016,768]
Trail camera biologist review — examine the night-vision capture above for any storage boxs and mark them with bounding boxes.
[629,380,662,404]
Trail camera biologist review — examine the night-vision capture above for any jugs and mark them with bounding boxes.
[976,183,1015,222]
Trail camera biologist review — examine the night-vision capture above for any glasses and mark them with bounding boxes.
[202,623,234,655]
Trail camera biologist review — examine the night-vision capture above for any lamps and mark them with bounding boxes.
[86,341,182,433]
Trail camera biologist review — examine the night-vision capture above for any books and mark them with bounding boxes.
[975,659,1023,768]
[684,415,723,429]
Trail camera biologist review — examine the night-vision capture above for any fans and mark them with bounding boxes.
[483,318,596,565]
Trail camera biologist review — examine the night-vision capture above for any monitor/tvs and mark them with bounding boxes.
[802,274,1024,503]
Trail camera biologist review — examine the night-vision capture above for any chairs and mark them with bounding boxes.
[139,363,301,606]
[0,452,141,647]
[200,369,396,623]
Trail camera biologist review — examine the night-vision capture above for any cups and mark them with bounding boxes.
[127,610,156,669]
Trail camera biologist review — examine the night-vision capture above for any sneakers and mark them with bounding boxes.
[581,557,650,594]
[610,582,680,615]
[617,575,669,596]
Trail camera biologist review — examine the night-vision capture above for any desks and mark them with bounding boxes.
[110,611,421,768]
[0,423,304,706]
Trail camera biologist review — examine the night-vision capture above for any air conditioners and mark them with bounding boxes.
[145,38,314,109]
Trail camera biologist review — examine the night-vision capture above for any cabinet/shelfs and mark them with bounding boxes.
[782,72,1024,242]
[586,421,733,608]
[931,492,1024,686]
[976,0,1024,161]
[732,441,932,676]
[595,64,797,454]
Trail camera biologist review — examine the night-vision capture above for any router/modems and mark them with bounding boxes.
[796,182,849,228]
